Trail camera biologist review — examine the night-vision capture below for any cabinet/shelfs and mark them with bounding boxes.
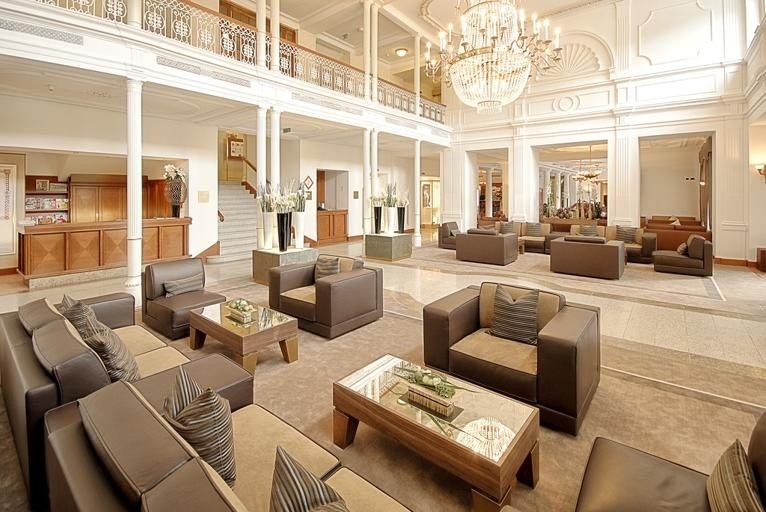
[69,173,180,224]
[23,173,70,225]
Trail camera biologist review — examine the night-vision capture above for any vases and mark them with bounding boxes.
[224,305,257,324]
[162,178,187,207]
[406,380,457,420]
[260,207,295,253]
[371,204,405,235]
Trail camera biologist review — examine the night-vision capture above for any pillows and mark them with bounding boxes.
[313,256,341,286]
[56,293,98,335]
[705,435,764,512]
[480,284,542,346]
[162,361,237,494]
[76,312,143,385]
[265,440,349,512]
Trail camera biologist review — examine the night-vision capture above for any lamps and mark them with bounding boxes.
[747,125,766,185]
[422,0,564,118]
[573,143,603,196]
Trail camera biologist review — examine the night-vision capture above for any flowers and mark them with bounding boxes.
[254,174,309,216]
[368,179,411,210]
[160,163,187,185]
[402,362,458,399]
[222,298,254,314]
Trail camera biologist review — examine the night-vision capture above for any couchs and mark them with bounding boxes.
[139,257,227,340]
[574,405,766,511]
[42,375,412,510]
[423,279,603,437]
[267,253,384,339]
[437,215,714,281]
[0,288,259,511]
